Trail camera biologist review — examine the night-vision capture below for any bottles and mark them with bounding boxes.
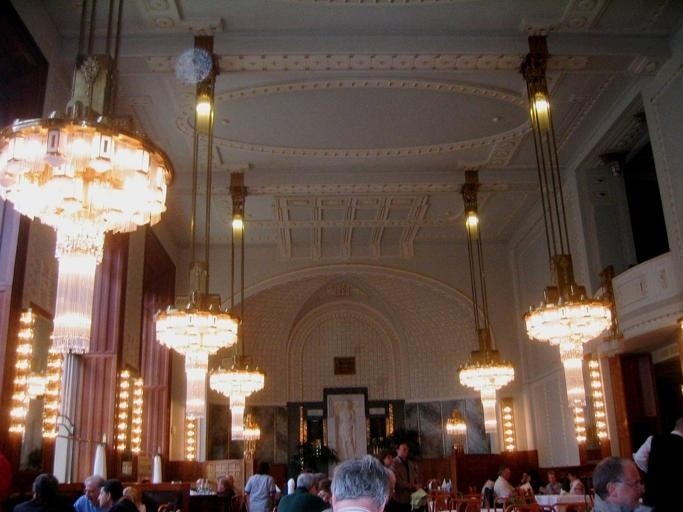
[193,477,210,495]
[428,477,452,489]
[286,477,294,494]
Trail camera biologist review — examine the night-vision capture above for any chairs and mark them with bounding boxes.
[412,475,595,512]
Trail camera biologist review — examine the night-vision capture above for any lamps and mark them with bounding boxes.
[444,409,467,453]
[151,34,241,420]
[458,169,512,434]
[515,35,613,410]
[210,172,267,437]
[0,0,174,357]
[238,412,261,457]
